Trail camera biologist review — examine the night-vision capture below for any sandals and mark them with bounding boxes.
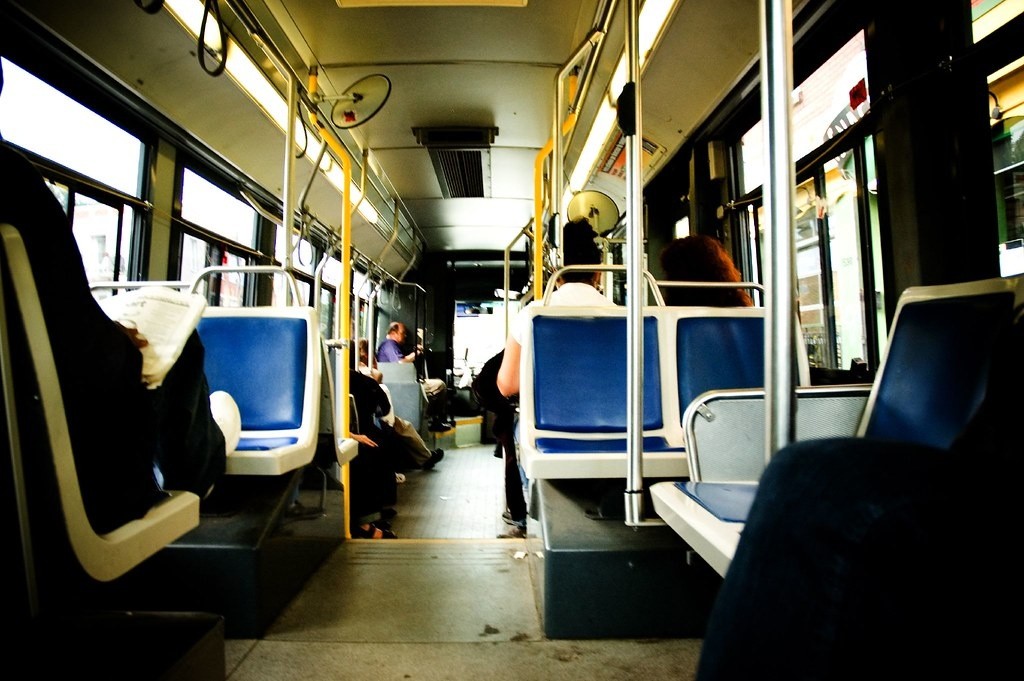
[353,523,398,540]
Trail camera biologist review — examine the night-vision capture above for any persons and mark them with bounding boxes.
[376,321,451,433]
[0,58,243,592]
[496,217,759,540]
[338,336,445,540]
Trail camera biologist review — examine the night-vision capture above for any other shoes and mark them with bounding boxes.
[420,447,444,471]
[281,500,325,519]
[427,422,451,433]
[380,507,397,517]
[502,511,526,526]
[496,527,527,538]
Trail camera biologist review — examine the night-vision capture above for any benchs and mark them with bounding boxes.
[0,222,430,679]
[517,260,1023,582]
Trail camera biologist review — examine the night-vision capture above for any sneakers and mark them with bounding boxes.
[202,391,242,498]
